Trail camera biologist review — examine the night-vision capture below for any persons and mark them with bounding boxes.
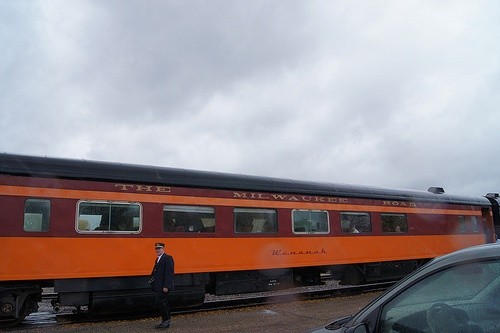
[93,211,409,234]
[146,242,175,329]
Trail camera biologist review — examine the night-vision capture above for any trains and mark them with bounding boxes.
[0,152,500,330]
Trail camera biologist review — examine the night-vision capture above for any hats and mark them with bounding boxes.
[154,242,165,249]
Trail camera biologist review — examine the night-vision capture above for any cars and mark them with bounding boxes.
[310,242,500,333]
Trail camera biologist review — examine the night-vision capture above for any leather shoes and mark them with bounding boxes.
[155,321,170,329]
